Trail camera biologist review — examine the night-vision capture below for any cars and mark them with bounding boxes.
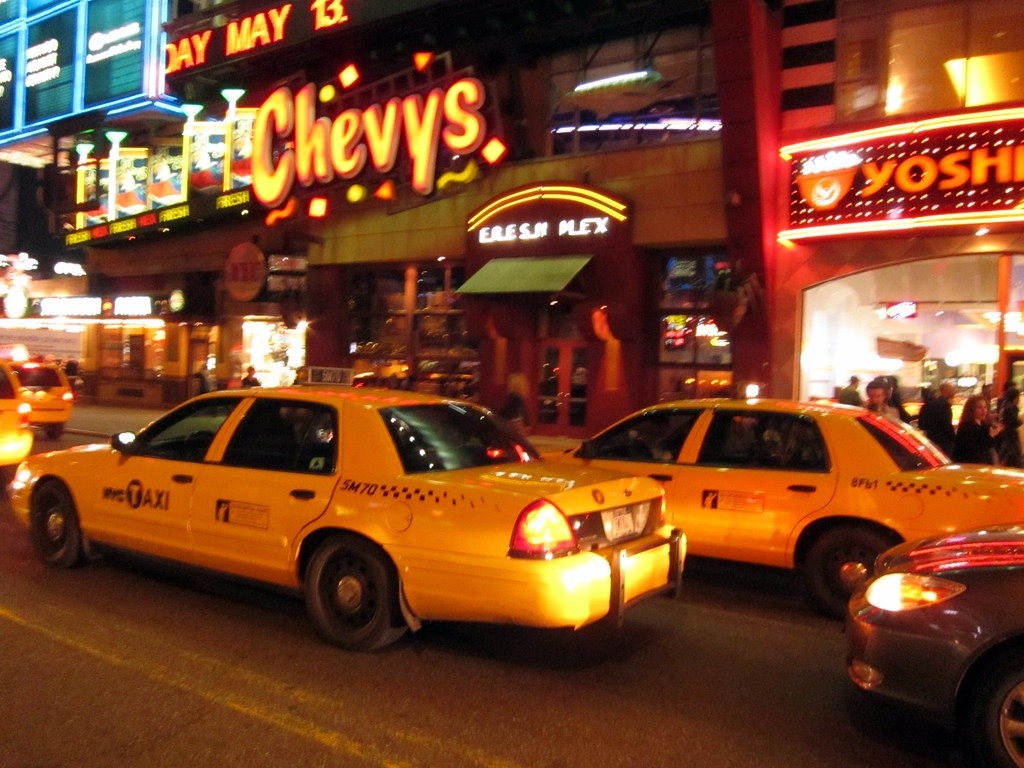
[7,386,683,654]
[0,363,34,467]
[844,523,1023,768]
[536,399,1024,619]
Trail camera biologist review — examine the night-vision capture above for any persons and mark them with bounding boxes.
[353,375,417,391]
[241,367,260,388]
[198,363,208,394]
[434,377,482,404]
[840,375,910,423]
[917,382,1024,468]
[650,421,695,460]
[499,393,532,431]
[29,352,80,386]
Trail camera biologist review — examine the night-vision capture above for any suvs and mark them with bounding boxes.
[1,358,77,440]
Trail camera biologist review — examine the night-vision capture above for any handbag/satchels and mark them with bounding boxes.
[509,417,526,439]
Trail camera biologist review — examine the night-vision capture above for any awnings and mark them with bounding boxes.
[256,265,377,310]
[0,274,218,323]
[456,254,595,292]
